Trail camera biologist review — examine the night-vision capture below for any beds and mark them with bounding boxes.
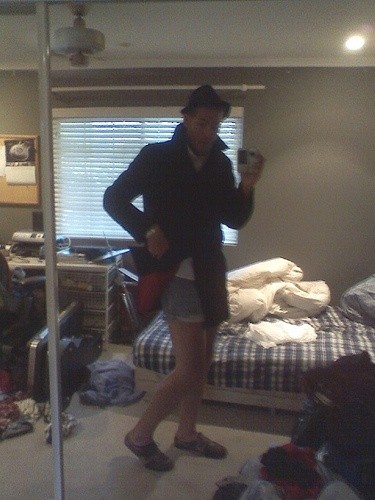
[132,257,375,411]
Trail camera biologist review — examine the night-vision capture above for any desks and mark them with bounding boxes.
[6,254,124,348]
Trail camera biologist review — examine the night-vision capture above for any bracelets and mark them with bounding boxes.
[145,229,155,240]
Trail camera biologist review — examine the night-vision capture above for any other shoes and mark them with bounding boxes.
[125,434,173,470]
[173,431,227,458]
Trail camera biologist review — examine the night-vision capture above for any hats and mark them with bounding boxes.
[179,84,229,116]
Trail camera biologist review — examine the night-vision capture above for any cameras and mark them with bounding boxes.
[238,150,257,173]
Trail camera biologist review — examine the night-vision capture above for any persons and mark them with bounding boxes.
[104,86,263,470]
[0,251,46,323]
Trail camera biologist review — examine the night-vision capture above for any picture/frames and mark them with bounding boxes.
[0,134,41,208]
